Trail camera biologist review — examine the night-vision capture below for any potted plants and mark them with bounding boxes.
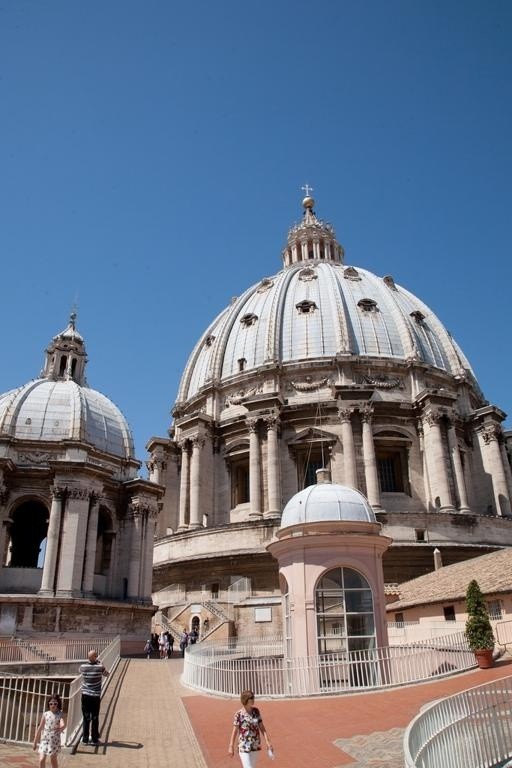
[464,579,496,668]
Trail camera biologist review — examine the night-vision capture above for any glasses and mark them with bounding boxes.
[248,696,254,699]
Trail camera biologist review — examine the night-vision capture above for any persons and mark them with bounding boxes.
[228,691,273,768]
[180,628,198,657]
[143,631,174,660]
[79,650,110,746]
[32,693,66,768]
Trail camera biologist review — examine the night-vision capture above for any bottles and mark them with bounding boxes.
[267,750,276,761]
